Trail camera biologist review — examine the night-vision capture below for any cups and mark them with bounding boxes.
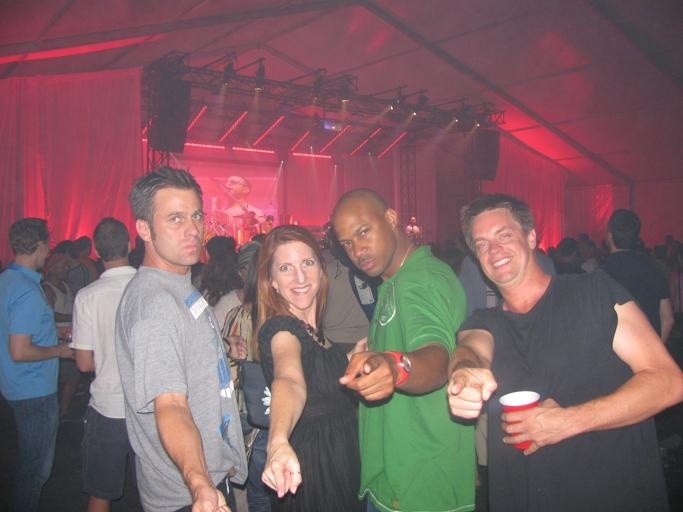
[499,391,541,450]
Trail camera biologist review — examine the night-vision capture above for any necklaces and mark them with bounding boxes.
[300,320,325,345]
[375,243,413,326]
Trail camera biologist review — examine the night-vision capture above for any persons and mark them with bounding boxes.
[0,166,681,511]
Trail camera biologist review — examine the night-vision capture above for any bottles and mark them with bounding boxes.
[64,328,72,345]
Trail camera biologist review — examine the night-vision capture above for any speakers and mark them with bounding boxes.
[477,128,501,180]
[148,65,192,153]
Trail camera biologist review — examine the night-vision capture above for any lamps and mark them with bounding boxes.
[203,60,483,129]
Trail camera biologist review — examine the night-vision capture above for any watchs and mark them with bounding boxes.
[383,352,411,385]
